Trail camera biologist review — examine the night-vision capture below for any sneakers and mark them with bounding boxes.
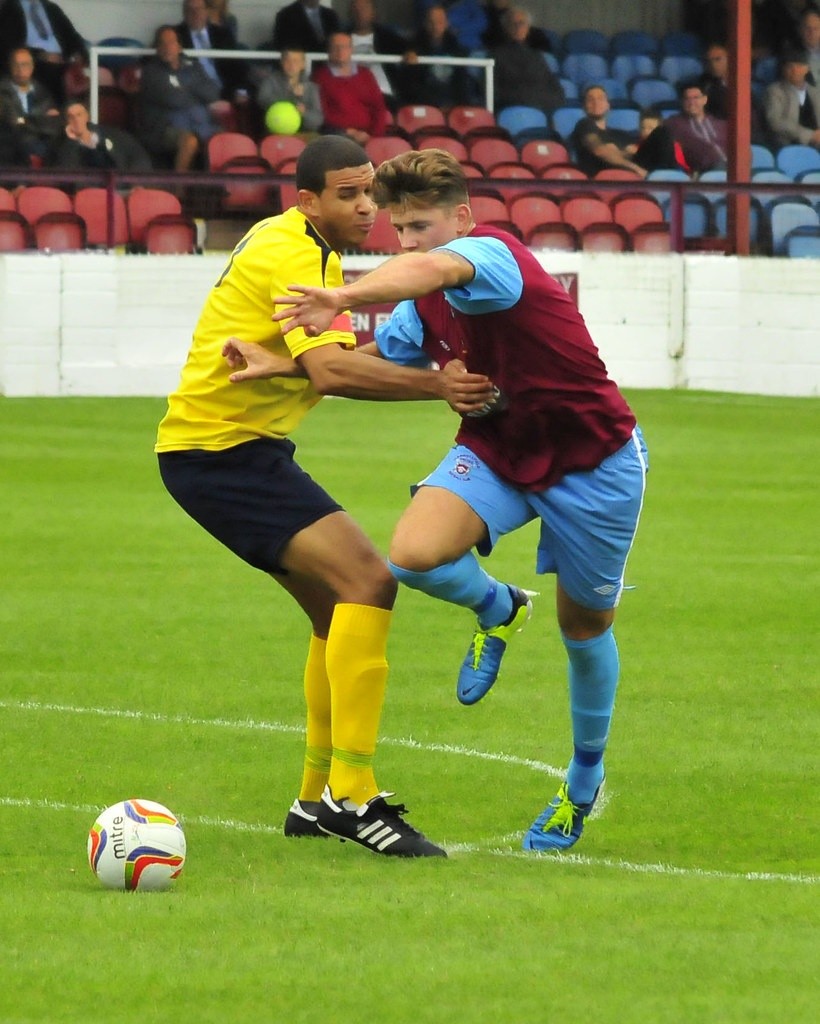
[524,761,605,851]
[316,784,447,859]
[455,582,534,707]
[284,798,346,842]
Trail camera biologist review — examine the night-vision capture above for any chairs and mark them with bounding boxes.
[0,19,820,254]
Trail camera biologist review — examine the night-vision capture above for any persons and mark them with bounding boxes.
[255,0,564,144]
[571,44,757,177]
[221,148,648,851]
[139,0,240,173]
[764,9,820,151]
[155,131,494,859]
[0,0,130,174]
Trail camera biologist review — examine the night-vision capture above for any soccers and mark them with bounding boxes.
[87,799,188,892]
